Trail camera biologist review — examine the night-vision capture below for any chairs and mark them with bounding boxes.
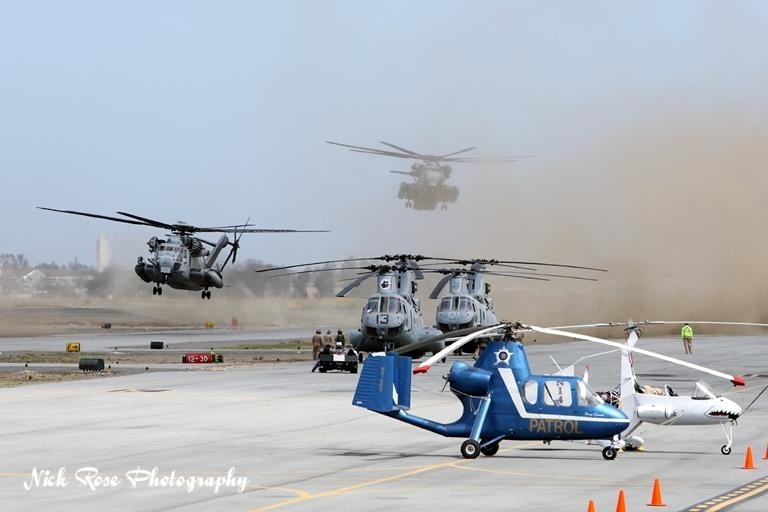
[664,384,679,397]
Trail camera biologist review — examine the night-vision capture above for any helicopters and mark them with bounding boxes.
[325,134,535,211]
[34,203,330,300]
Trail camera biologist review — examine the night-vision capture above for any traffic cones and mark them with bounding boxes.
[741,447,757,470]
[615,489,627,512]
[586,498,597,512]
[646,479,667,507]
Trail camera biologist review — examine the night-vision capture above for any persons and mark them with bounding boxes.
[681,323,694,354]
[335,329,345,348]
[311,329,323,360]
[346,343,358,356]
[312,344,331,372]
[323,329,333,346]
